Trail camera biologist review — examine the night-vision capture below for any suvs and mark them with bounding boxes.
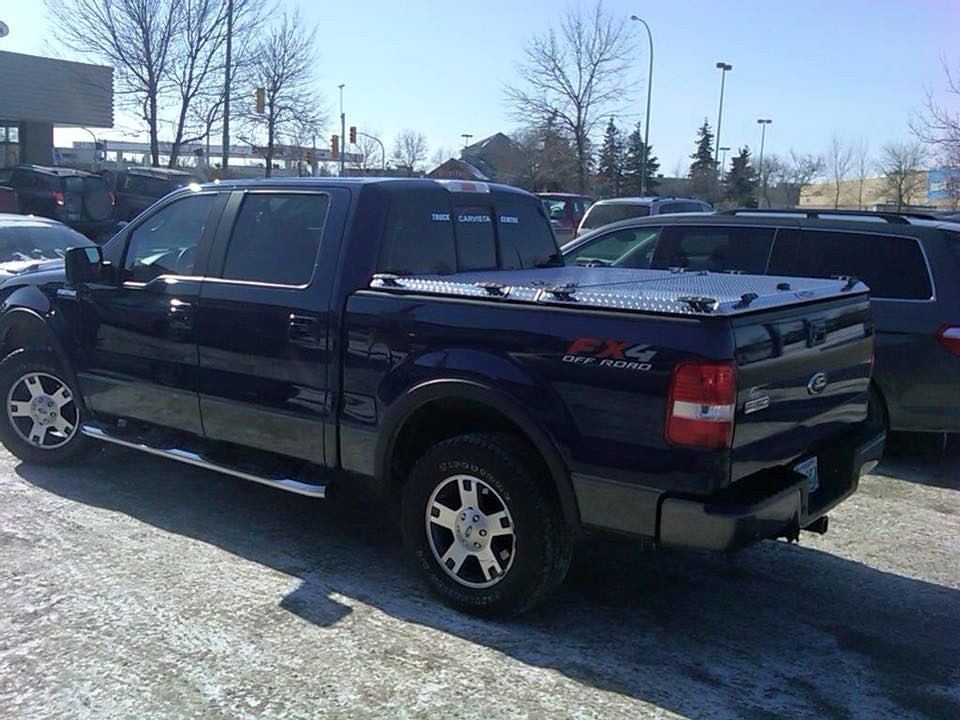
[93,164,204,235]
[575,193,718,258]
[532,192,597,248]
[558,204,960,435]
[1,162,116,233]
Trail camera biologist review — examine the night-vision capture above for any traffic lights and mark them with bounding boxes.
[349,126,356,144]
[305,149,311,164]
[330,135,339,156]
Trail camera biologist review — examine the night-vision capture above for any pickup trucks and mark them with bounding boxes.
[1,177,888,624]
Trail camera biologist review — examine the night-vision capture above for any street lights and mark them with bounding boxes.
[630,14,654,197]
[713,61,734,171]
[756,119,773,187]
[337,84,345,177]
[462,134,473,163]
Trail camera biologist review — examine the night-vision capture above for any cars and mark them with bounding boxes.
[0,213,101,284]
